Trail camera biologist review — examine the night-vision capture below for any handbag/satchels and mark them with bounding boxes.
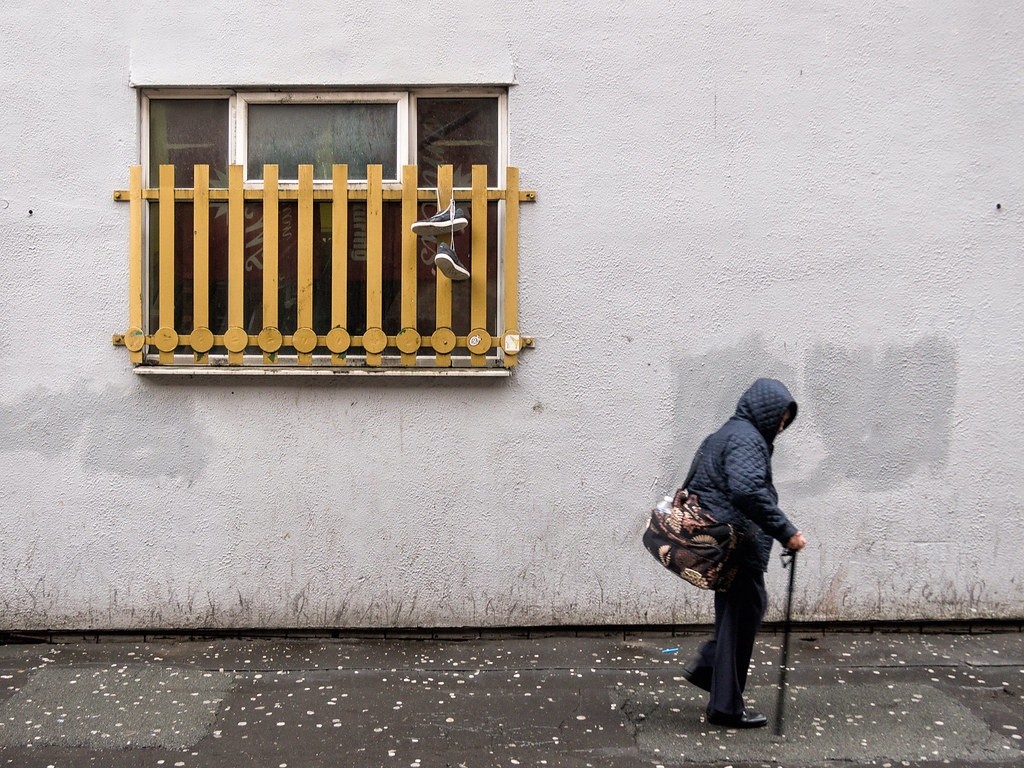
[643,489,739,589]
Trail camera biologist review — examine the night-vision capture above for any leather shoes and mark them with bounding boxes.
[706,708,768,728]
[682,653,712,692]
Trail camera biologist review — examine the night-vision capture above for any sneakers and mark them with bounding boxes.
[434,242,470,279]
[412,209,468,237]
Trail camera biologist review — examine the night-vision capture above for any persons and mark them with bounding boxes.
[642,377,807,728]
